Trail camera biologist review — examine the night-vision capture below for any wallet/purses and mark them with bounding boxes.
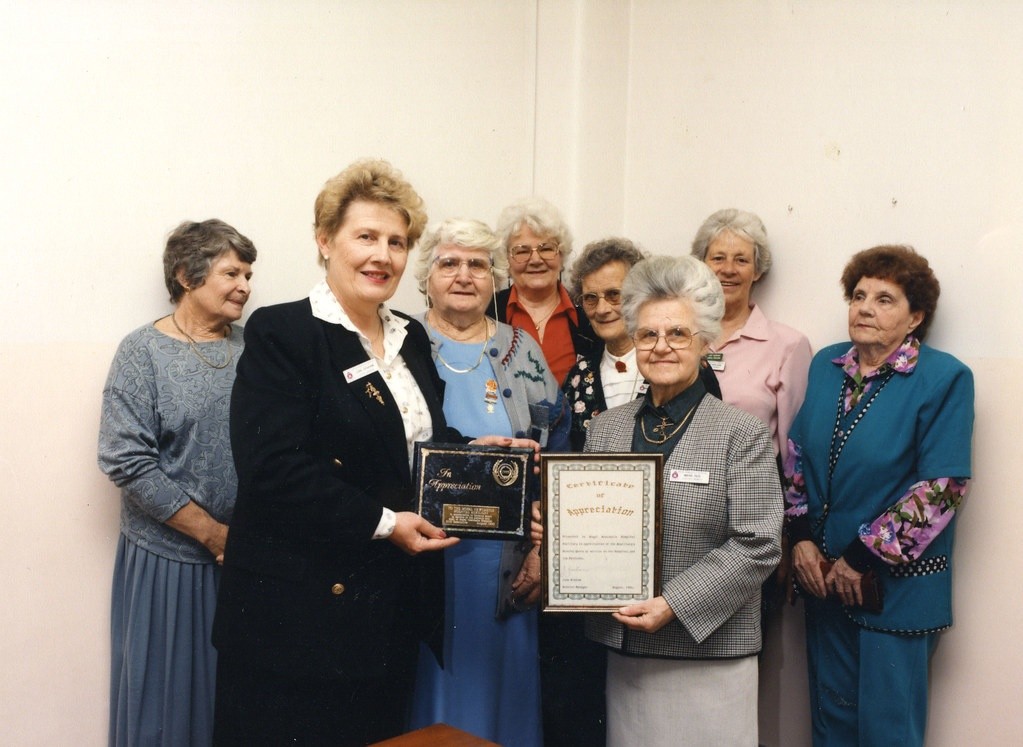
[819,560,885,615]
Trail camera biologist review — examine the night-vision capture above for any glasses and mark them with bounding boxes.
[430,251,496,279]
[508,242,561,263]
[575,288,625,310]
[628,326,703,351]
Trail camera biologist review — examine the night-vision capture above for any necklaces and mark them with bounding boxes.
[172,312,232,369]
[427,311,489,374]
[535,302,558,331]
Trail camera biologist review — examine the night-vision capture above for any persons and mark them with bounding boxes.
[484,197,594,388]
[99,218,257,747]
[690,209,813,747]
[530,256,784,747]
[210,158,541,747]
[561,238,723,747]
[408,217,572,747]
[781,246,974,747]
